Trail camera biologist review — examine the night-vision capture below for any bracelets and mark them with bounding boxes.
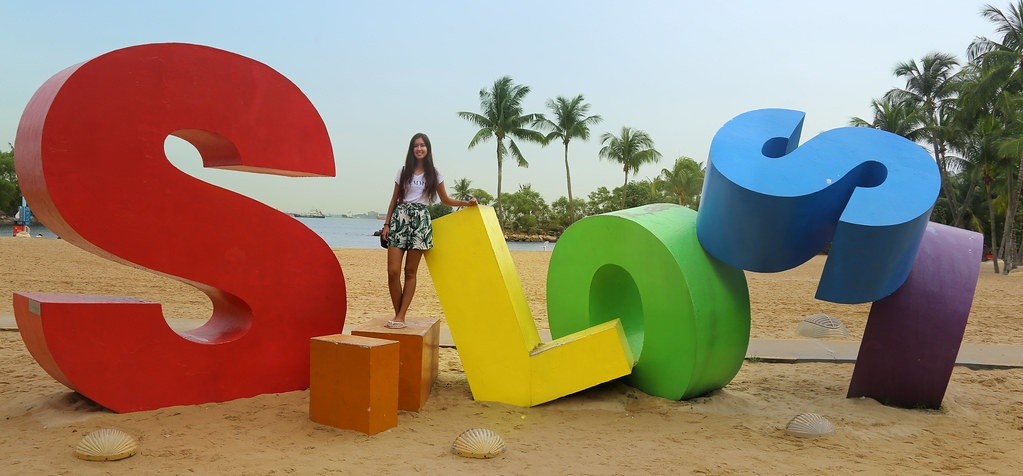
[384,224,389,225]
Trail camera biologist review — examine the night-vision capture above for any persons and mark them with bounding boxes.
[382,133,478,328]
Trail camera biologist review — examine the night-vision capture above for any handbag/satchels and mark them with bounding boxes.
[380,228,389,249]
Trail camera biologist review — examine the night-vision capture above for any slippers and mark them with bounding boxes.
[387,321,408,328]
[384,320,392,327]
